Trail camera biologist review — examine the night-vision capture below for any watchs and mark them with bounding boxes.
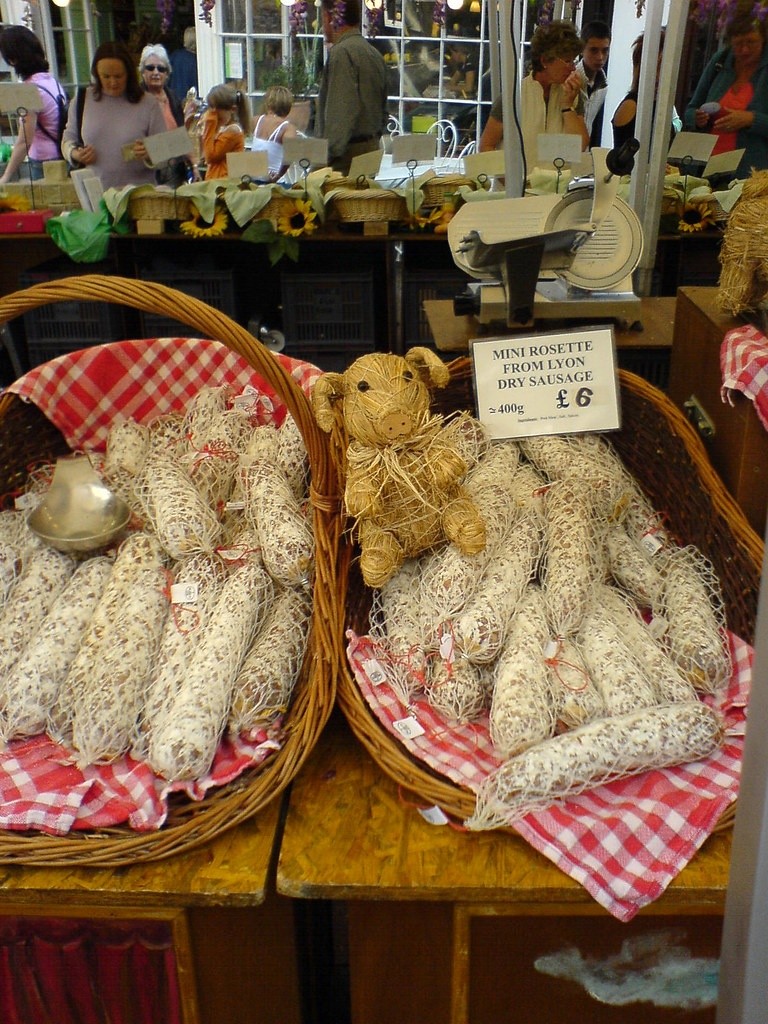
[561,106,575,113]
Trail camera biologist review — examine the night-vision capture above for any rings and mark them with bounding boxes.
[573,87,578,91]
[727,126,730,129]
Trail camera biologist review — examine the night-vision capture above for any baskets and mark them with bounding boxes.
[663,183,739,221]
[0,276,342,866]
[336,353,766,841]
[130,192,192,221]
[322,176,410,221]
[251,193,296,222]
[416,176,477,210]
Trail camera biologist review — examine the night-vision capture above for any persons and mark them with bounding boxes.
[445,43,478,92]
[478,21,590,152]
[611,32,677,175]
[202,84,251,181]
[61,42,167,192]
[169,27,199,100]
[0,26,68,183]
[251,86,296,179]
[313,0,388,180]
[564,21,611,151]
[139,44,201,190]
[683,14,768,192]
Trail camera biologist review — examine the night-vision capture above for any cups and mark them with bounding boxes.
[121,140,141,162]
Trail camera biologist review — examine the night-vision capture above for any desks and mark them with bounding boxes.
[0,786,298,1024]
[275,700,735,1024]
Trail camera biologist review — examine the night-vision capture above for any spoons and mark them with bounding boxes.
[26,435,132,554]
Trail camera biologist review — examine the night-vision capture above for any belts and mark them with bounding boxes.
[347,130,381,143]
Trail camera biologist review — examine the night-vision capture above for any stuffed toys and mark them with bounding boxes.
[315,347,487,588]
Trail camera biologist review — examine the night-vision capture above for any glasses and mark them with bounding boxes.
[144,64,167,73]
[558,58,578,68]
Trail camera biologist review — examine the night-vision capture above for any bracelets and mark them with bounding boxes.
[192,164,197,168]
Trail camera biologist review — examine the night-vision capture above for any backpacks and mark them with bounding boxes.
[32,80,69,153]
